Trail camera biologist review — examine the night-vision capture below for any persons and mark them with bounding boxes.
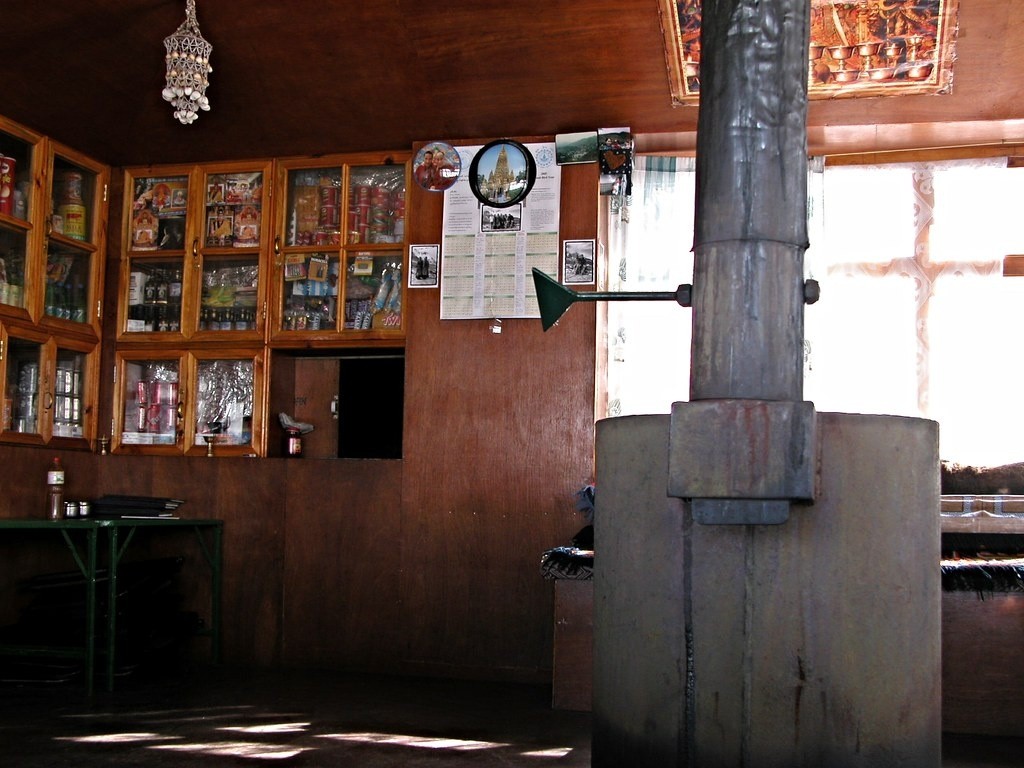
[433,150,456,189]
[604,136,623,150]
[612,176,626,195]
[488,211,516,229]
[416,253,428,280]
[414,151,434,188]
[573,252,585,274]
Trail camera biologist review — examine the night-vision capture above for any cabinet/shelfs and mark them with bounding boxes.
[0,114,412,461]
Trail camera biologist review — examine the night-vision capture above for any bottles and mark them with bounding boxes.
[198,306,255,330]
[45,457,64,520]
[60,165,83,205]
[44,277,86,323]
[129,268,182,332]
[79,501,90,516]
[65,502,76,516]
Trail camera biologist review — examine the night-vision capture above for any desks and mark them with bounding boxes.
[0,517,225,706]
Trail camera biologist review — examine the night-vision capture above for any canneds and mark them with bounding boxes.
[135,379,178,435]
[310,185,406,247]
[14,363,81,438]
[281,427,301,458]
[0,157,85,242]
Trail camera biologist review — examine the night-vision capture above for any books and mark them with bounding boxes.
[85,493,185,521]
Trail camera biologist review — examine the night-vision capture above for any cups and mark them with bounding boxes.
[0,153,16,217]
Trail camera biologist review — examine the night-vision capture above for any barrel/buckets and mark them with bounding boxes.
[57,204,87,241]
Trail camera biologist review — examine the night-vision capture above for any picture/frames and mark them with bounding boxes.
[659,1,959,104]
[471,139,536,208]
